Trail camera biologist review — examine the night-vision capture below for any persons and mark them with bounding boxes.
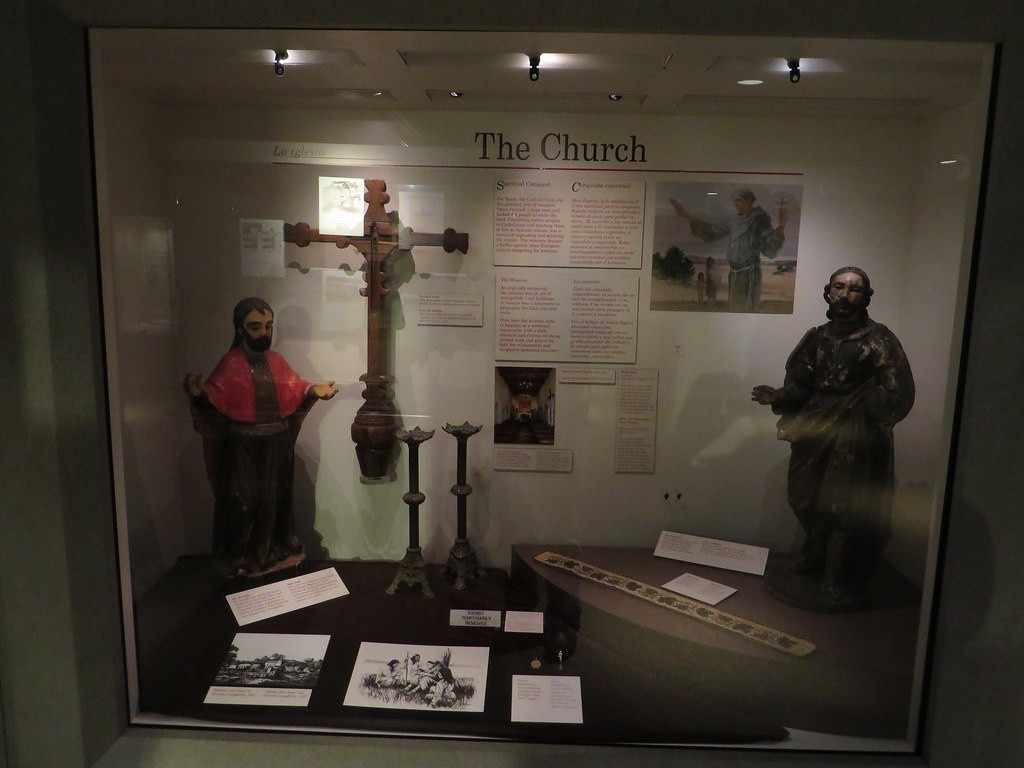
[751,266,916,600]
[183,296,338,580]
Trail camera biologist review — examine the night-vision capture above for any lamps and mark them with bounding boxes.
[529,59,540,81]
[274,53,288,76]
[789,61,800,83]
